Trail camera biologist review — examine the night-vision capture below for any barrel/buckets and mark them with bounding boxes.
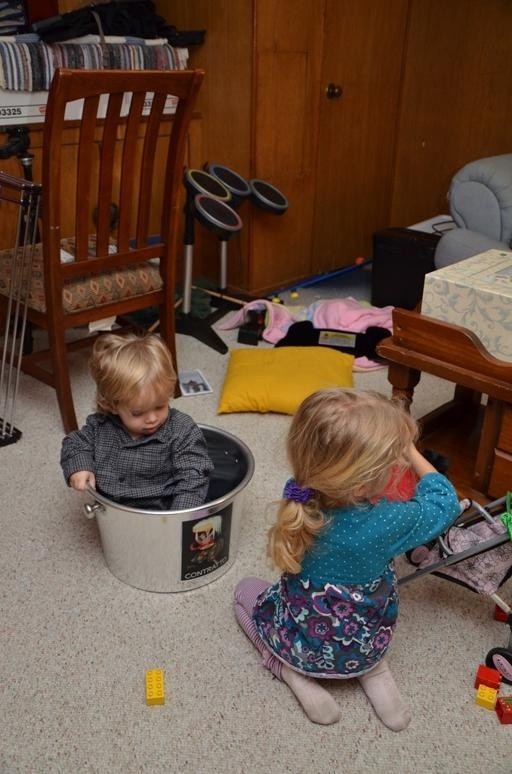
[84,424,254,592]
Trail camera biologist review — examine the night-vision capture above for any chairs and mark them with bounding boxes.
[0,67,206,435]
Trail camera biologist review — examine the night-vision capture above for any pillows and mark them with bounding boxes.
[216,347,354,415]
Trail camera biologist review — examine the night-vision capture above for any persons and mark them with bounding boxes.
[61,331,213,510]
[233,386,462,732]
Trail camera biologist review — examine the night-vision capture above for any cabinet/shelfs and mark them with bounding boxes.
[0,114,201,315]
[158,0,410,298]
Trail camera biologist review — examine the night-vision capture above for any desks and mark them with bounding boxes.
[377,308,511,526]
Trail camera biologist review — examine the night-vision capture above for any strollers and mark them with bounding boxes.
[397,492,512,685]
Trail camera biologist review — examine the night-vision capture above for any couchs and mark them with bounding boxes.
[434,154,512,268]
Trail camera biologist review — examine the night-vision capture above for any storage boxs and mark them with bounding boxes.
[420,249,512,364]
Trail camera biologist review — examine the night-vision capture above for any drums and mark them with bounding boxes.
[191,193,241,240]
[182,169,232,205]
[211,165,251,205]
[252,178,289,216]
